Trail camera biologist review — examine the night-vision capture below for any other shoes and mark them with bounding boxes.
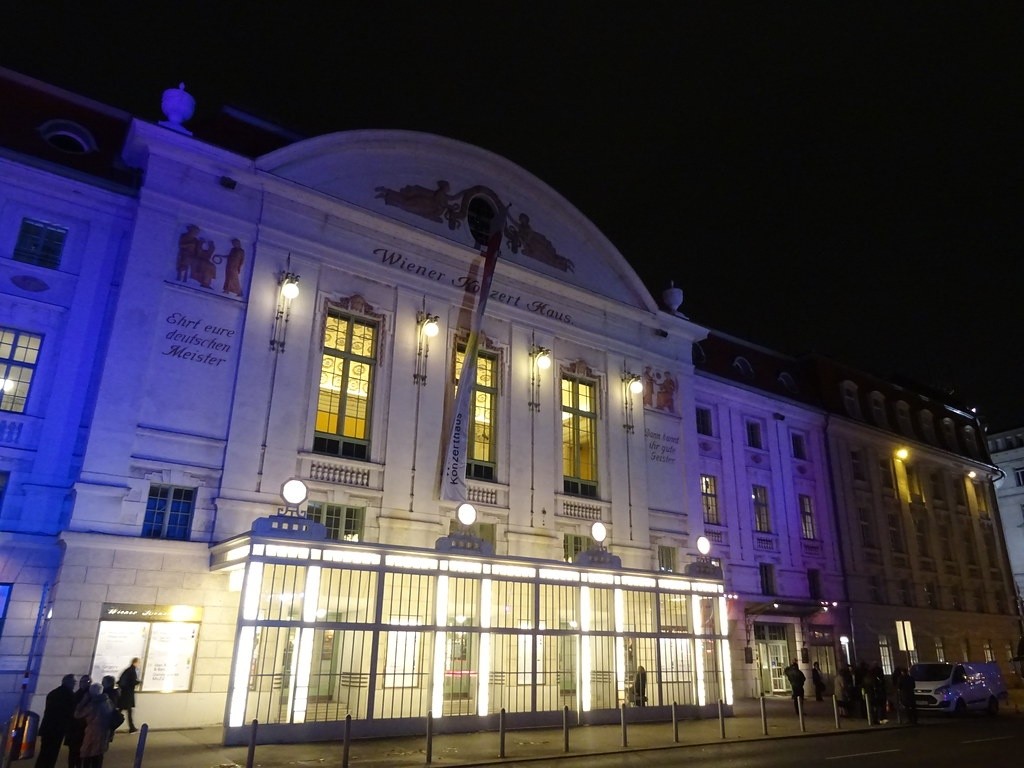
[904,720,918,725]
[843,715,847,718]
[872,720,880,724]
[817,700,824,702]
[882,713,888,719]
[803,712,806,716]
[839,714,843,718]
[129,729,140,734]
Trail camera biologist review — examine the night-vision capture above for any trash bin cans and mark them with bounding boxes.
[5,711,39,760]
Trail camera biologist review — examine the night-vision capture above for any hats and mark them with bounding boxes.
[89,684,103,695]
[80,674,92,683]
[61,674,78,683]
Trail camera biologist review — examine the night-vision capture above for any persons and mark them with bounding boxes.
[812,661,826,703]
[630,666,647,705]
[34,656,142,768]
[833,661,918,725]
[784,661,807,714]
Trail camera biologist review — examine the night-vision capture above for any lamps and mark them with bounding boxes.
[271,271,300,354]
[590,521,607,550]
[696,536,712,564]
[529,346,551,412]
[413,311,439,386]
[622,372,644,435]
[456,503,477,537]
[278,477,309,518]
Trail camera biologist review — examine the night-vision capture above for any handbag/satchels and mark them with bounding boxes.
[820,681,826,693]
[111,709,124,732]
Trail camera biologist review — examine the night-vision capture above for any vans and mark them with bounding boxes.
[909,661,1007,718]
